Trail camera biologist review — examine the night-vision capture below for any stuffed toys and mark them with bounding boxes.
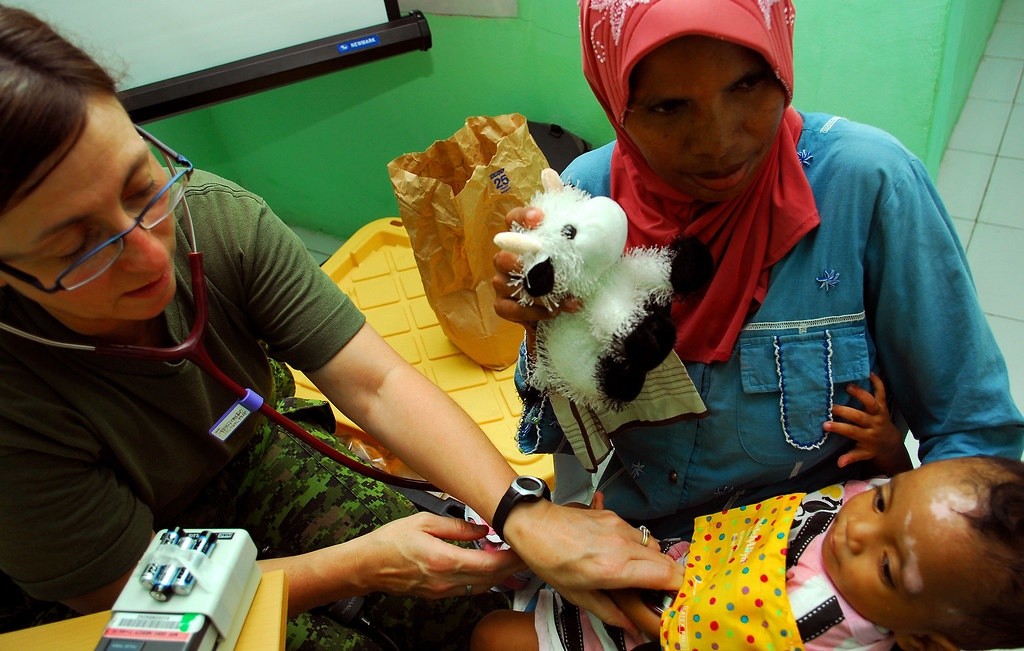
[494,168,714,414]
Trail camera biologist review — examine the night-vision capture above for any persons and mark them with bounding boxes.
[0,8,686,651]
[471,372,1024,651]
[493,0,1024,520]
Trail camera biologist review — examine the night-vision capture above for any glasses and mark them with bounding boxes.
[0,122,193,295]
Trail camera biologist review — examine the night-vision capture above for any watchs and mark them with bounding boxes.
[492,476,551,545]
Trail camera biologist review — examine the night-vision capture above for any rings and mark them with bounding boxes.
[466,585,472,592]
[638,525,650,546]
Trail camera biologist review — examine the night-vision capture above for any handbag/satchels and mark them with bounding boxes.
[526,120,591,175]
